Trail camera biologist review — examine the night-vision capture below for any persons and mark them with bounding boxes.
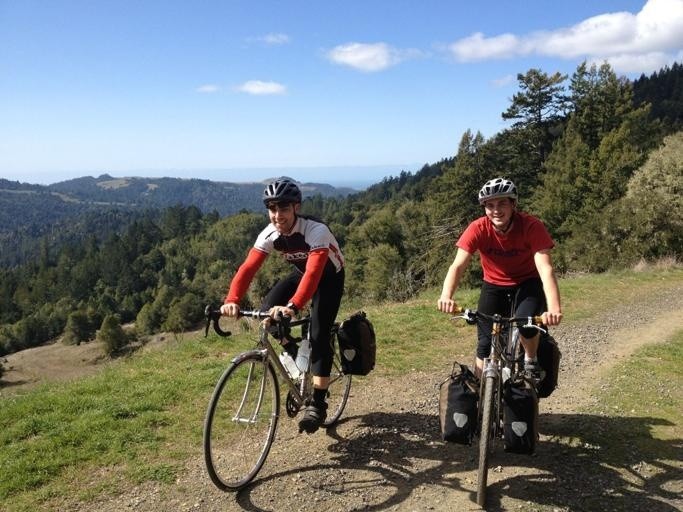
[218,180,345,434]
[436,176,564,394]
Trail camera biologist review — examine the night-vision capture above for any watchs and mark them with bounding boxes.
[286,302,299,315]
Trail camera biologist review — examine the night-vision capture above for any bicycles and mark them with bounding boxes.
[203,305,354,492]
[437,302,562,506]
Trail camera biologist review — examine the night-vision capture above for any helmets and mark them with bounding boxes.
[262,179,301,207]
[477,177,518,205]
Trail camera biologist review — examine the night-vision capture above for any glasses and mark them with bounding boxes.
[266,199,291,209]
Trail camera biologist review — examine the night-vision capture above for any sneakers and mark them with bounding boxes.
[523,360,539,386]
[298,402,327,433]
[280,349,298,373]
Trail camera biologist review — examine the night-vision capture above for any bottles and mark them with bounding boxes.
[295,339,311,371]
[279,351,301,381]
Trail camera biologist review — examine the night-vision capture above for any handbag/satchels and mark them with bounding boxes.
[517,323,561,400]
[437,362,479,445]
[337,313,377,376]
[501,376,539,455]
